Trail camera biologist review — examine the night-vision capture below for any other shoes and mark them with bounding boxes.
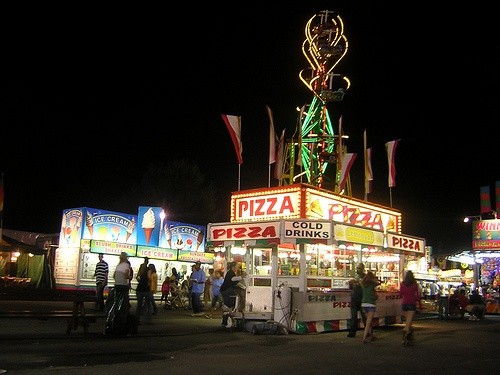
[152,311,158,315]
[362,337,380,343]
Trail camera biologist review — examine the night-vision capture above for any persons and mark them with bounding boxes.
[348,263,381,344]
[92,252,248,326]
[417,280,484,321]
[400,270,419,346]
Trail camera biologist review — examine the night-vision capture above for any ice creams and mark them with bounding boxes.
[164,223,172,248]
[196,228,204,251]
[125,221,134,242]
[86,210,93,235]
[74,214,82,231]
[62,213,66,239]
[141,208,156,245]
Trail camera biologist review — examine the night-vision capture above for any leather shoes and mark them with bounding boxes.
[347,333,356,337]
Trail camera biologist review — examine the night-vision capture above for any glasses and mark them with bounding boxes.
[356,267,361,269]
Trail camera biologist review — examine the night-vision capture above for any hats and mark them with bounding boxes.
[191,265,199,270]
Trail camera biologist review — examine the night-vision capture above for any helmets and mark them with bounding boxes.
[196,261,201,265]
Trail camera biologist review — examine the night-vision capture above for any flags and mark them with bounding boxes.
[221,115,242,165]
[267,106,276,164]
[275,129,284,181]
[339,153,357,193]
[365,148,373,193]
[385,140,396,187]
[364,130,368,177]
